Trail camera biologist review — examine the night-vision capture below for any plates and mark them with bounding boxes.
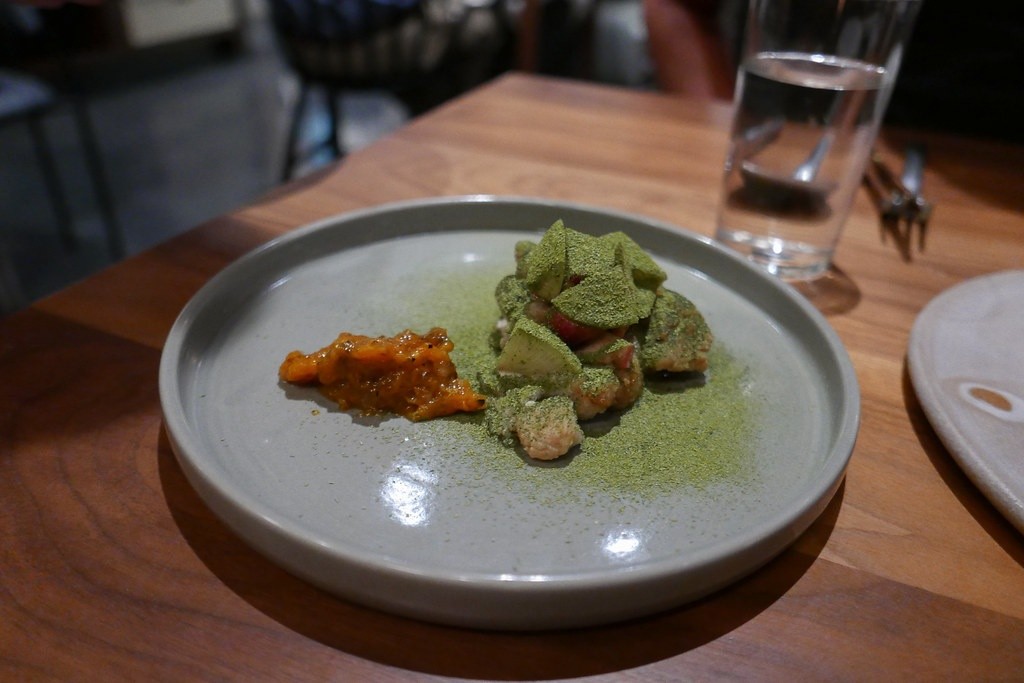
[157,193,863,627]
[908,269,1024,536]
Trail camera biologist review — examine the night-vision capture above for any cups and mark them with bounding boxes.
[714,49,893,283]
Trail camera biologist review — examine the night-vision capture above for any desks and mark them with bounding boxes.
[0,72,1024,683]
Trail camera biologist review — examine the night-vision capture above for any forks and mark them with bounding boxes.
[881,148,936,226]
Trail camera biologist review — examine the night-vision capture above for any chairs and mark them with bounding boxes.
[0,0,129,261]
[271,0,380,183]
